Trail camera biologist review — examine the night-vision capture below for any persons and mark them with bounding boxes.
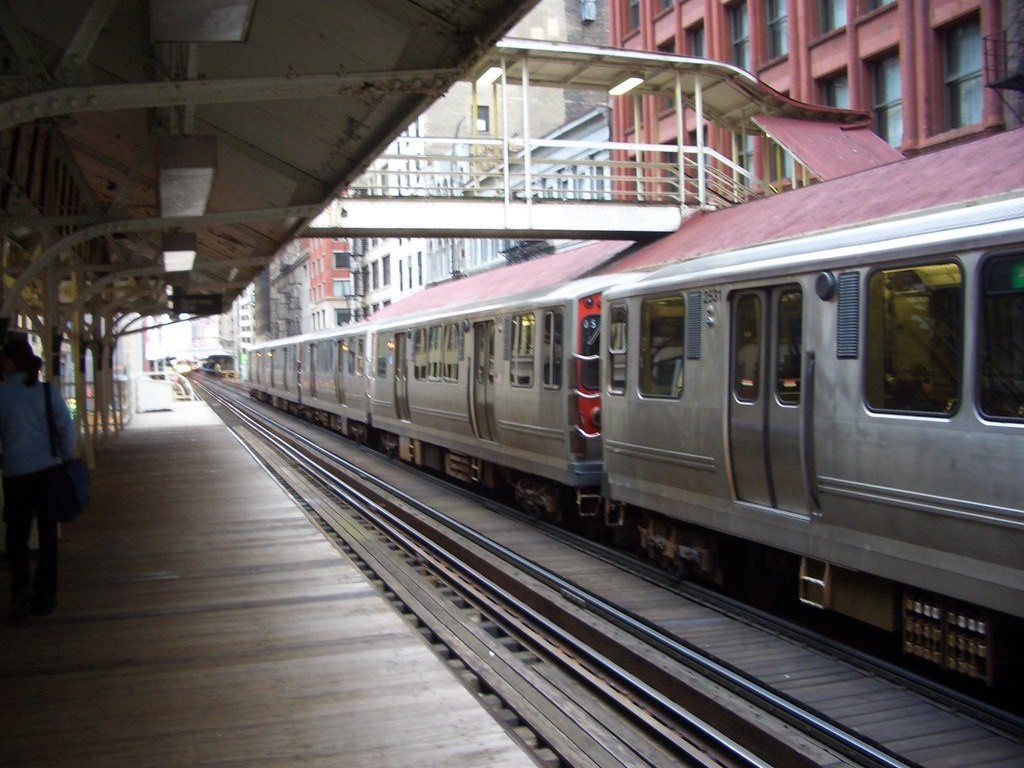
[890,363,928,410]
[737,329,757,378]
[0,340,78,623]
[214,362,221,377]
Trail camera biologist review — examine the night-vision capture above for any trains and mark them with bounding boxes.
[242,129,1024,686]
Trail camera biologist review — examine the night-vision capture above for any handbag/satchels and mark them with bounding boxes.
[52,456,87,514]
